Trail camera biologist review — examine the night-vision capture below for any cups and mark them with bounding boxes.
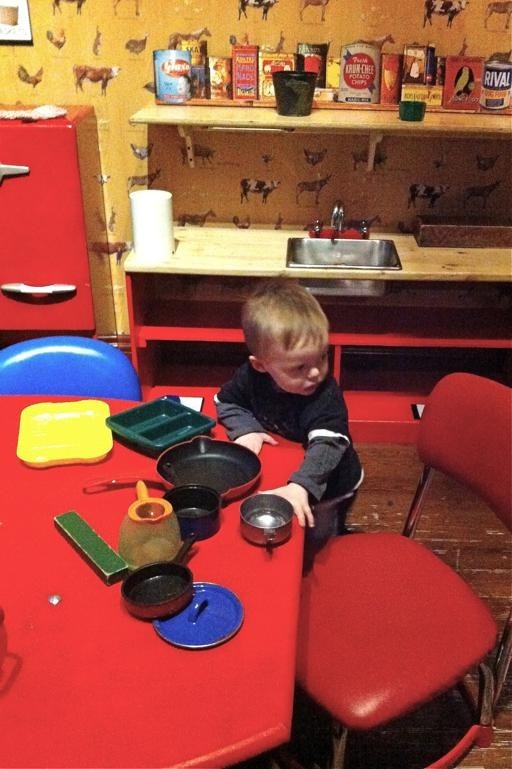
[271,70,318,117]
[398,100,426,121]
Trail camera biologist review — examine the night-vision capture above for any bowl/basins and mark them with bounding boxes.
[161,484,221,541]
[239,494,293,554]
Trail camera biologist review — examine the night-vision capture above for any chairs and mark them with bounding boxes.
[0,335,143,402]
[296,373,511,769]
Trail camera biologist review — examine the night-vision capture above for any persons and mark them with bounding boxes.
[213,279,364,533]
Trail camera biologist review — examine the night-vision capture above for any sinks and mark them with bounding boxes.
[286,237,402,270]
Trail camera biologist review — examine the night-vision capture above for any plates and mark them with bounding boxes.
[15,398,113,468]
[105,396,216,453]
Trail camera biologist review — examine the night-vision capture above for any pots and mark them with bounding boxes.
[84,434,263,502]
[120,530,199,619]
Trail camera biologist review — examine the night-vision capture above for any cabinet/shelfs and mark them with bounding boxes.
[122,227,511,444]
[0,105,117,336]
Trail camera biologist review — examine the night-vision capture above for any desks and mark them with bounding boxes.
[0,393,307,769]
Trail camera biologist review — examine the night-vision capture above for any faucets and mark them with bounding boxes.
[330,200,345,232]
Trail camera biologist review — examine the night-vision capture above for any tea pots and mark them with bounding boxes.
[118,480,181,562]
